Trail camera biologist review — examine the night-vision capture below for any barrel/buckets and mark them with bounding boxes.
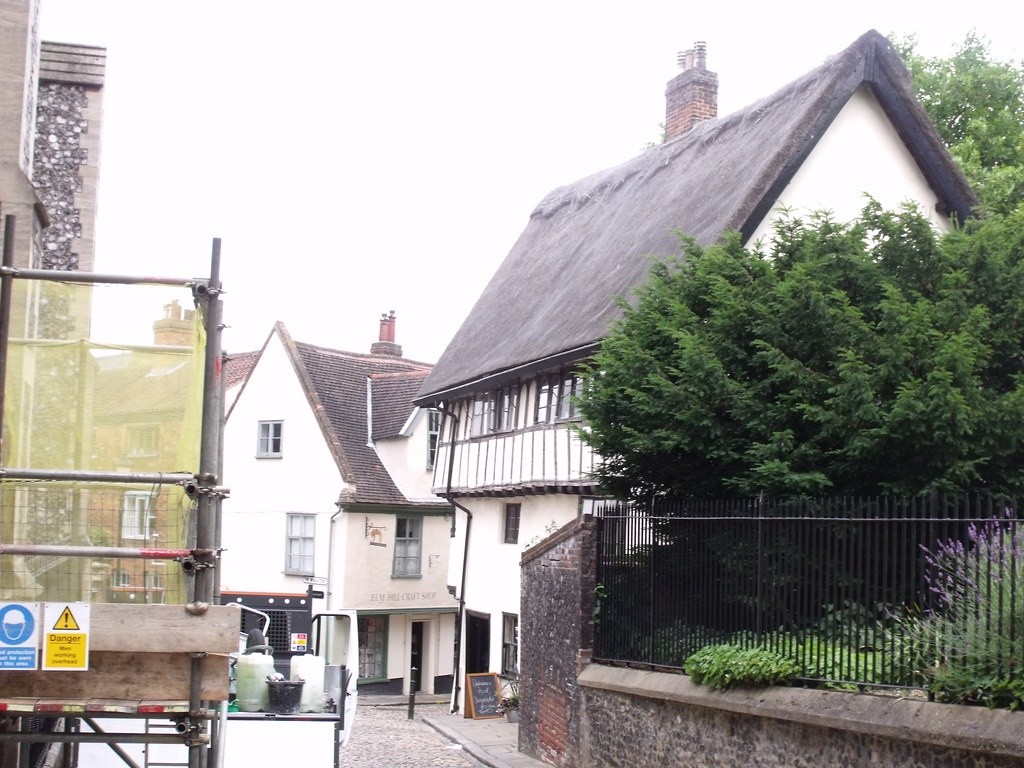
[266,680,304,714]
[236,652,274,712]
[289,653,325,712]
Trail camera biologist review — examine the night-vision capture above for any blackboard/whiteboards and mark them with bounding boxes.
[463,672,504,720]
